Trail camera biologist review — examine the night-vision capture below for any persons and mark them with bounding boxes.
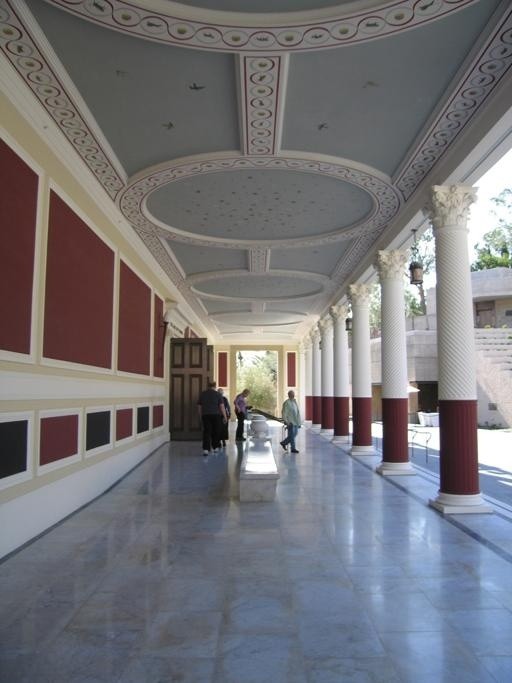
[234,389,252,441]
[197,381,231,455]
[280,391,301,453]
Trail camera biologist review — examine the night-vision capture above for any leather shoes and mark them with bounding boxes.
[236,437,246,441]
[280,442,287,451]
[291,448,299,453]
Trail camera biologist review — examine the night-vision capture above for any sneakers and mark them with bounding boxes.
[213,448,219,453]
[202,449,209,456]
